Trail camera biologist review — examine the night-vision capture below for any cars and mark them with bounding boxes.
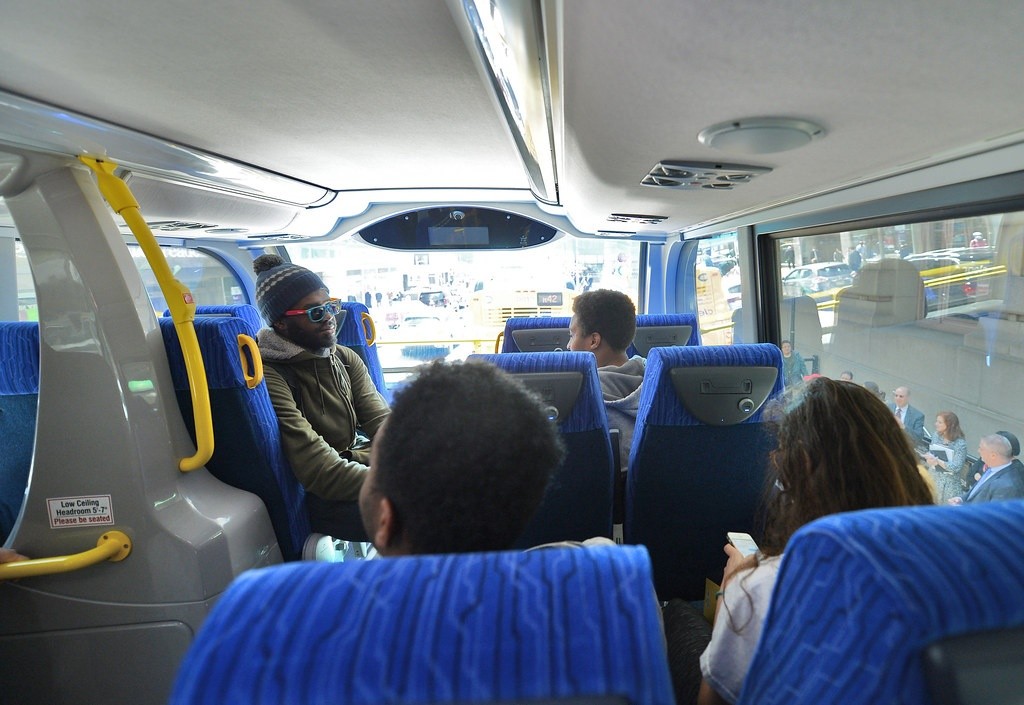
[372,274,637,384]
[696,246,1009,344]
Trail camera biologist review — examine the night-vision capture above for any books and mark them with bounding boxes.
[929,443,954,476]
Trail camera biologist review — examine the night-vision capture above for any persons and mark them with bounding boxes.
[840,371,853,382]
[848,246,861,271]
[970,232,988,247]
[253,254,392,541]
[916,464,940,504]
[810,245,821,262]
[614,253,632,280]
[887,386,925,446]
[895,240,909,258]
[782,340,810,390]
[856,240,865,257]
[862,382,886,401]
[920,411,967,505]
[833,248,845,262]
[567,288,645,477]
[945,431,1024,504]
[661,376,936,705]
[785,245,795,269]
[360,356,568,559]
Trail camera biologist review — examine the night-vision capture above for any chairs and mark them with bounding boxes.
[804,271,810,278]
[0,302,1024,705]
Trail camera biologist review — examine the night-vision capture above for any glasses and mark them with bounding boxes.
[893,393,906,400]
[283,296,344,323]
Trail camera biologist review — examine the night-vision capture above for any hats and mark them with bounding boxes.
[252,254,330,327]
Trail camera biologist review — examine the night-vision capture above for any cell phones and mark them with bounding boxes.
[726,532,766,560]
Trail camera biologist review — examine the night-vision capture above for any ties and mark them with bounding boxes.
[895,409,901,420]
[967,467,992,500]
